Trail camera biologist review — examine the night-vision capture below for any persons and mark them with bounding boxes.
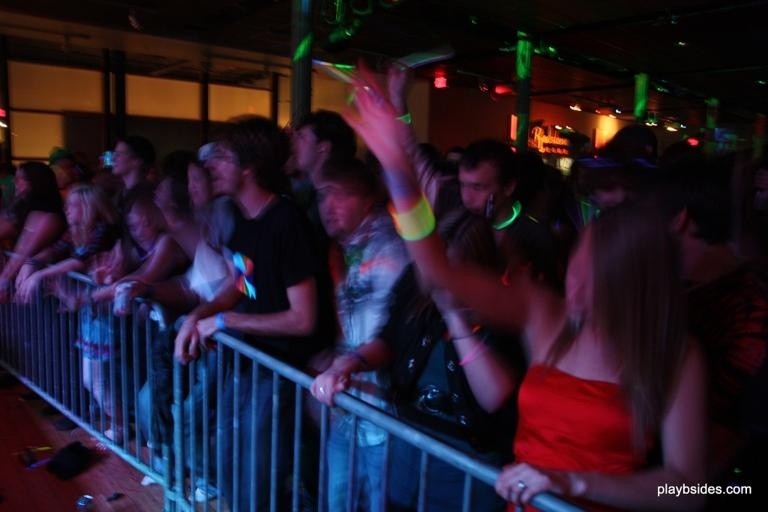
[1,55,766,509]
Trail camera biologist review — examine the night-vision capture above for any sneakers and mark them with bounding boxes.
[185,488,224,504]
[55,412,90,432]
[139,465,176,488]
